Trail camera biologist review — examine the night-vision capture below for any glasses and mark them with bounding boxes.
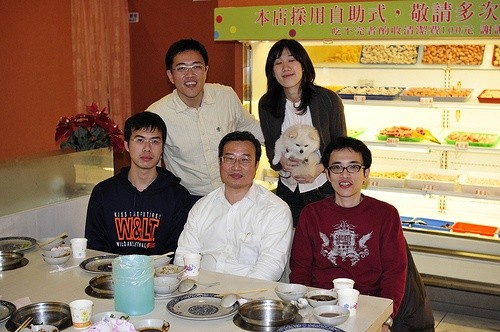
[130,136,164,146]
[327,164,365,174]
[220,155,257,167]
[171,63,207,74]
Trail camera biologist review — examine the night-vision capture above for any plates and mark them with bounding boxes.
[155,284,196,300]
[0,236,37,253]
[166,292,240,321]
[0,300,16,322]
[79,255,120,274]
[272,322,345,332]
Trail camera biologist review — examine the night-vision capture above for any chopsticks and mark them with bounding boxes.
[13,316,33,332]
[213,289,267,297]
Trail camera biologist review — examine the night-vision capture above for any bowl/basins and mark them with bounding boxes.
[89,274,115,295]
[134,319,170,332]
[276,283,350,326]
[154,276,182,295]
[156,265,185,278]
[236,299,299,326]
[37,237,72,264]
[10,302,71,329]
[0,251,24,271]
[91,311,131,325]
[150,255,171,268]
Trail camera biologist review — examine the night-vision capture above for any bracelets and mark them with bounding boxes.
[385,317,394,328]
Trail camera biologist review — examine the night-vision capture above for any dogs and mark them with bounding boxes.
[271,123,321,181]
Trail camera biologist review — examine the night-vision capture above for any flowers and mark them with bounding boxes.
[55,102,124,153]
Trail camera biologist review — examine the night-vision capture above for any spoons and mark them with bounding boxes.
[220,294,264,308]
[179,279,220,291]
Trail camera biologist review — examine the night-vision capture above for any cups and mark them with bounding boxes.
[112,254,154,316]
[333,278,355,292]
[68,299,94,329]
[337,288,360,317]
[70,238,88,258]
[21,325,59,332]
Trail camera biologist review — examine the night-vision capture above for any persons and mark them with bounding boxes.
[84,112,196,264]
[258,38,346,229]
[173,130,294,282]
[289,138,408,332]
[141,38,265,218]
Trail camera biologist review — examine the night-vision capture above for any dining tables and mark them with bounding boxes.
[0,249,393,332]
